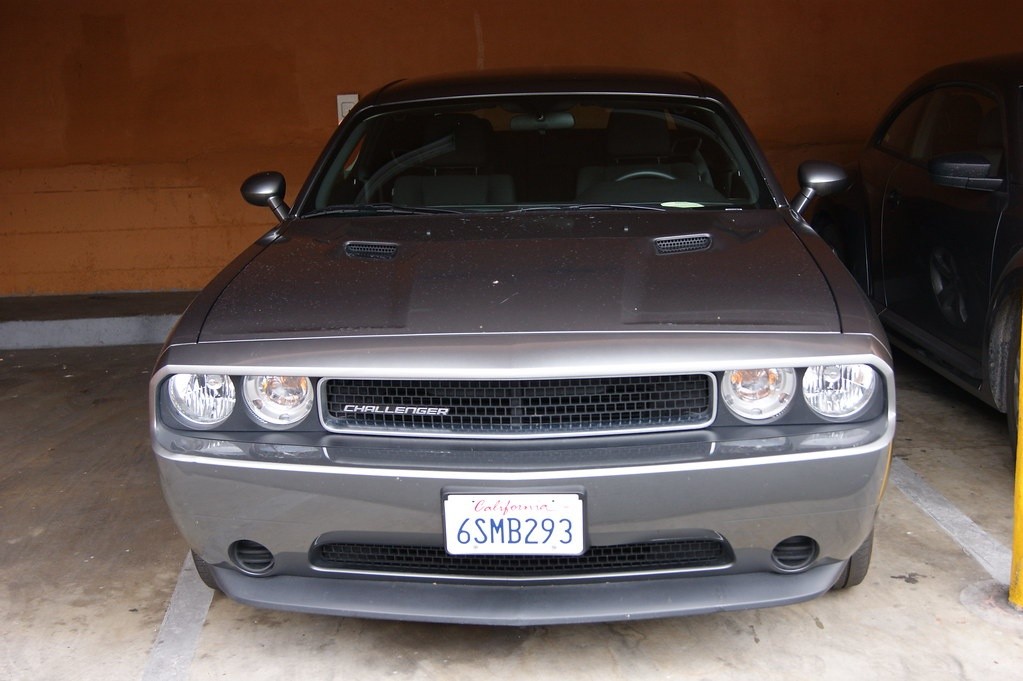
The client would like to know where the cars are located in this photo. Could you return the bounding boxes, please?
[146,64,897,629]
[809,52,1023,474]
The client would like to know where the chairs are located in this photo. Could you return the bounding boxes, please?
[376,114,515,206]
[575,108,706,205]
